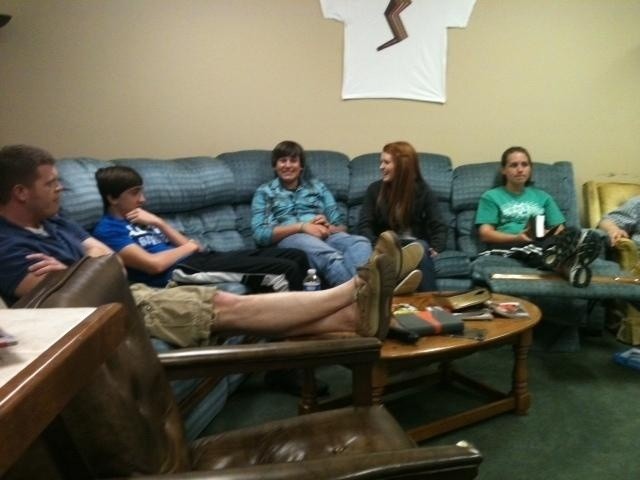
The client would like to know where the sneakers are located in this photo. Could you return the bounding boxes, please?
[394,269,422,297]
[542,226,580,269]
[355,254,393,340]
[369,231,404,281]
[568,229,602,288]
[399,241,425,279]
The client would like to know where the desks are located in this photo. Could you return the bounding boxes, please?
[0,291,131,480]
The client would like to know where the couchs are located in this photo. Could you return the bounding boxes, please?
[348,152,471,294]
[584,180,639,346]
[451,160,639,300]
[15,254,483,480]
[51,158,229,438]
[112,156,273,387]
[216,150,348,291]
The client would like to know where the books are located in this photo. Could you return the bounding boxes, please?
[390,310,465,339]
[452,300,531,321]
[522,214,546,240]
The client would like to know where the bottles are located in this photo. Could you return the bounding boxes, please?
[304,269,321,290]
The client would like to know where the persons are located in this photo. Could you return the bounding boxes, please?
[249,139,425,295]
[0,145,403,349]
[356,140,448,292]
[596,193,640,247]
[474,146,603,290]
[91,165,329,399]
